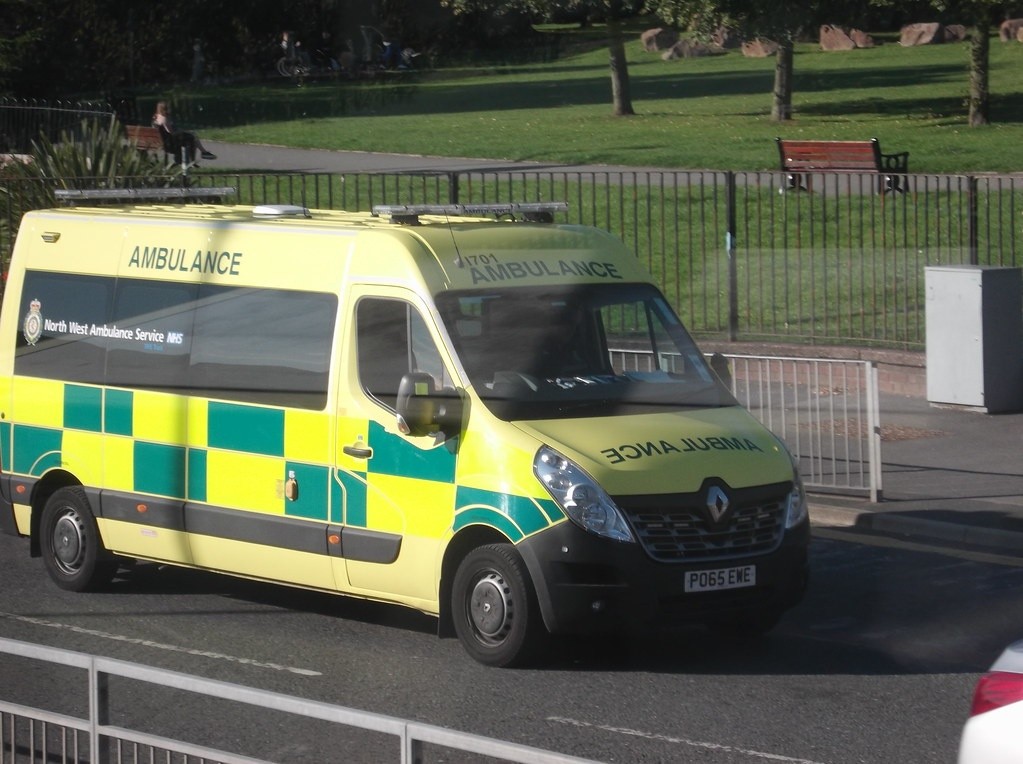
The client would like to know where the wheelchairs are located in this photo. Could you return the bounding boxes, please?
[276,49,318,78]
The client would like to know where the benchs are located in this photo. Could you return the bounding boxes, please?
[776,137,910,197]
[127,125,188,165]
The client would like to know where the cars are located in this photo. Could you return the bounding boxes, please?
[955,640,1023,764]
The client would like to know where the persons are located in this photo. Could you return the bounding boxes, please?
[152,102,217,169]
[541,317,614,380]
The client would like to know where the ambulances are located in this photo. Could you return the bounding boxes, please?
[0,204,812,667]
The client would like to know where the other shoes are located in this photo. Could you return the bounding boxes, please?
[191,164,200,169]
[200,150,217,159]
